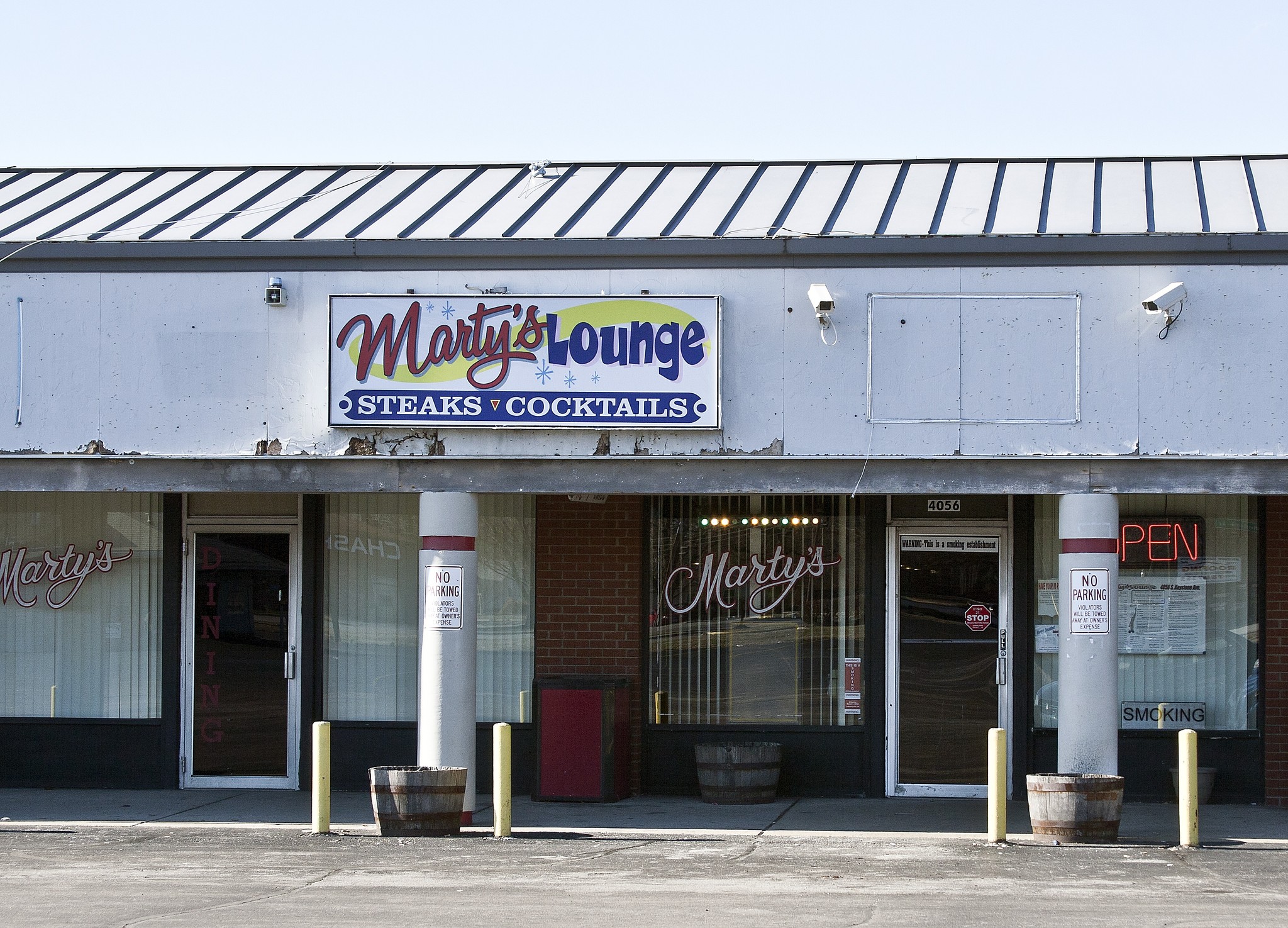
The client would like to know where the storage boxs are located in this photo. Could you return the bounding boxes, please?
[535,678,629,803]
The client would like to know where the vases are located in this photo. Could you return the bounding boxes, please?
[1169,767,1216,804]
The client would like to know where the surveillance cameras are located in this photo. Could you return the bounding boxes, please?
[808,281,835,323]
[1139,281,1187,315]
